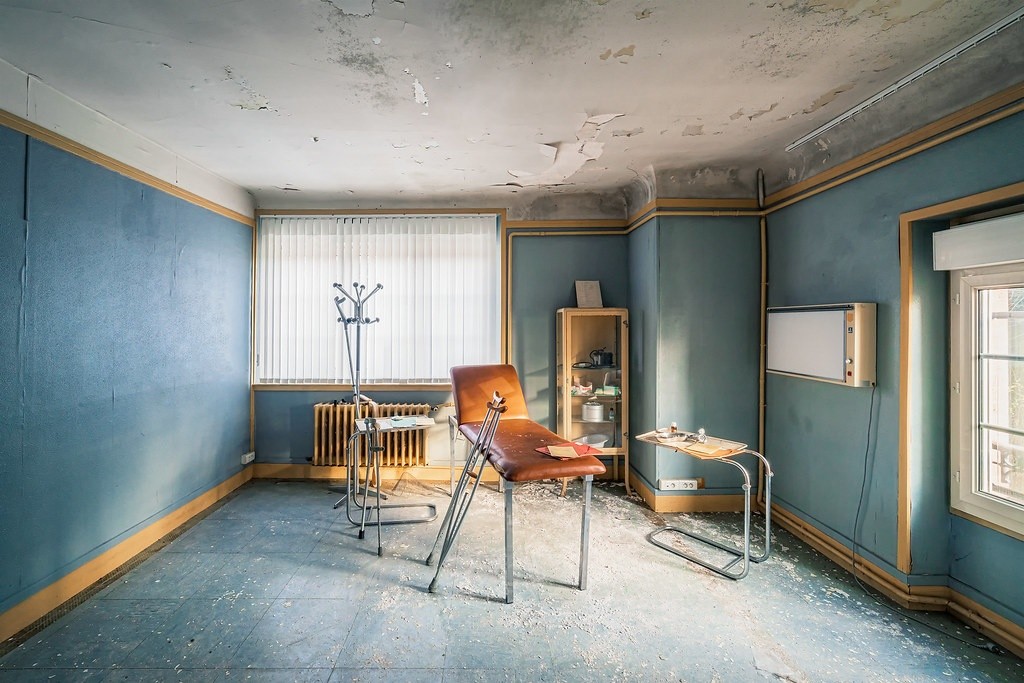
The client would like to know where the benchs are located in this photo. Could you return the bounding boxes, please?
[449,363,607,604]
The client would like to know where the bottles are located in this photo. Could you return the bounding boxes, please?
[669,421,678,435]
[697,428,707,443]
[609,408,615,421]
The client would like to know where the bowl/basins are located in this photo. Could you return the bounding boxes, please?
[573,434,609,448]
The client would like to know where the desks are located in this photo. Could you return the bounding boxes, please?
[634,427,774,579]
[345,414,439,525]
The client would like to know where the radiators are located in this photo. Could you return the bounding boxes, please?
[314,402,431,468]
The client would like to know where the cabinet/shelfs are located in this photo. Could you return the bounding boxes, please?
[556,307,634,500]
